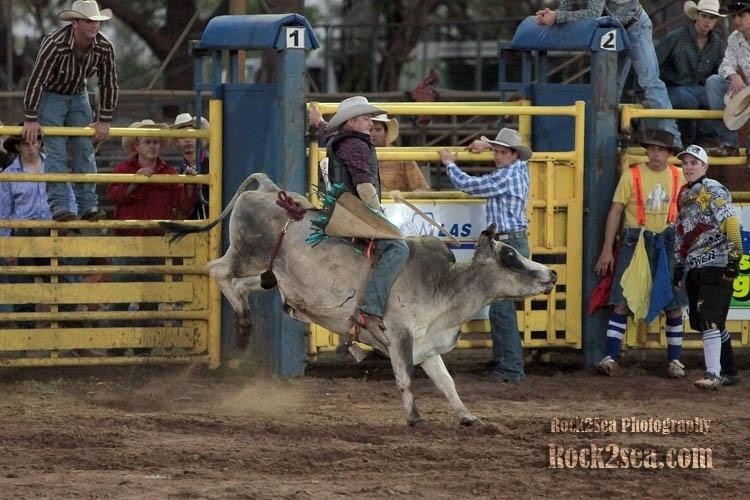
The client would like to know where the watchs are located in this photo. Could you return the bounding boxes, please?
[316,118,324,129]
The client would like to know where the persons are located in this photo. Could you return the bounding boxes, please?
[438,127,532,383]
[592,127,688,379]
[306,95,410,347]
[673,144,744,390]
[629,0,728,146]
[308,103,429,190]
[23,0,120,222]
[169,112,209,220]
[706,0,750,170]
[535,0,682,149]
[0,122,89,357]
[108,118,199,356]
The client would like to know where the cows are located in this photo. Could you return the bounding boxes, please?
[157,173,557,432]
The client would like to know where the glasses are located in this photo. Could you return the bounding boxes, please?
[724,1,745,12]
[136,137,161,147]
[487,144,512,154]
[17,139,41,148]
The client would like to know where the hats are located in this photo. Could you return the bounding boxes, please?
[323,94,387,133]
[369,113,400,146]
[2,122,44,154]
[480,127,533,161]
[719,0,750,17]
[169,112,210,149]
[675,143,710,166]
[638,128,683,156]
[56,1,113,22]
[120,119,169,154]
[683,1,730,21]
[722,85,750,132]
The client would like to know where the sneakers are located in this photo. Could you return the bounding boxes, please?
[349,308,392,347]
[720,370,742,387]
[471,361,528,383]
[591,355,619,377]
[666,357,686,380]
[707,142,742,159]
[693,373,724,391]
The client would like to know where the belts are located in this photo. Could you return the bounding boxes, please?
[624,6,644,30]
[494,230,529,241]
